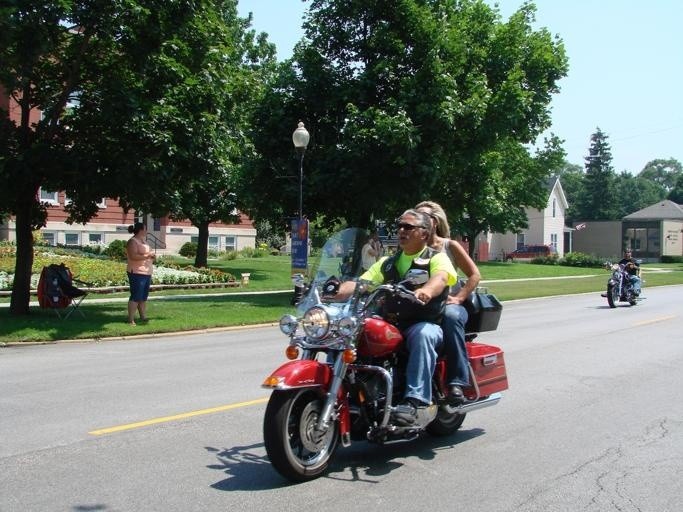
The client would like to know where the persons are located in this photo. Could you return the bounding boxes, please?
[415,200,482,402]
[600,248,641,302]
[360,235,380,274]
[124,221,157,327]
[337,255,353,282]
[290,208,458,427]
[375,240,384,262]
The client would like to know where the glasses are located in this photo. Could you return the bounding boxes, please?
[397,224,425,230]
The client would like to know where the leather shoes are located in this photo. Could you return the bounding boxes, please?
[394,401,419,426]
[449,385,465,403]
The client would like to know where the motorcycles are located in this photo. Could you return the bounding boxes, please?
[605,259,646,308]
[261,226,508,482]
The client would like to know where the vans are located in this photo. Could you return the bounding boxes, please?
[506,245,559,264]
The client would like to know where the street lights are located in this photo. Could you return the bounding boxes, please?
[292,118,310,220]
